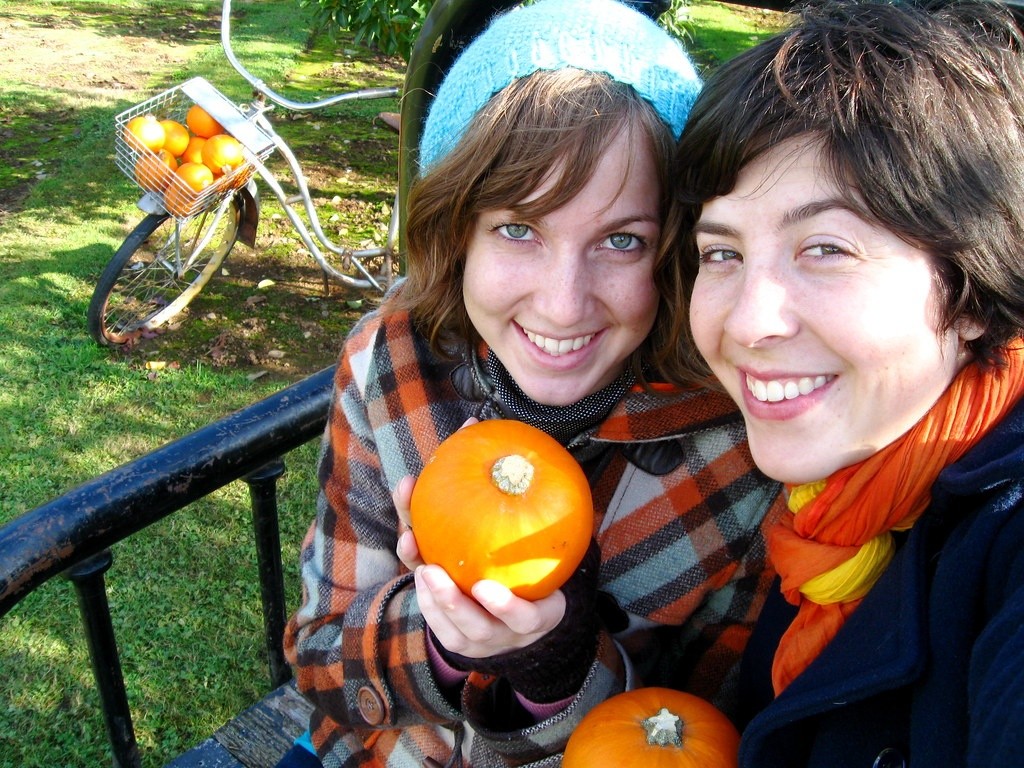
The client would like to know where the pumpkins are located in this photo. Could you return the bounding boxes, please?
[562,686,738,768]
[123,103,250,218]
[410,421,593,603]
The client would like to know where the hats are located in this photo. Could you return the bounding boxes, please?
[414,0,706,182]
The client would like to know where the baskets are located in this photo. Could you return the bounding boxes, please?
[114,77,280,225]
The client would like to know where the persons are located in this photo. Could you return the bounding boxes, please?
[661,0,1024,768]
[273,0,789,768]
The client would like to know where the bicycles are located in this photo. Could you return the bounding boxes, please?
[86,0,409,347]
